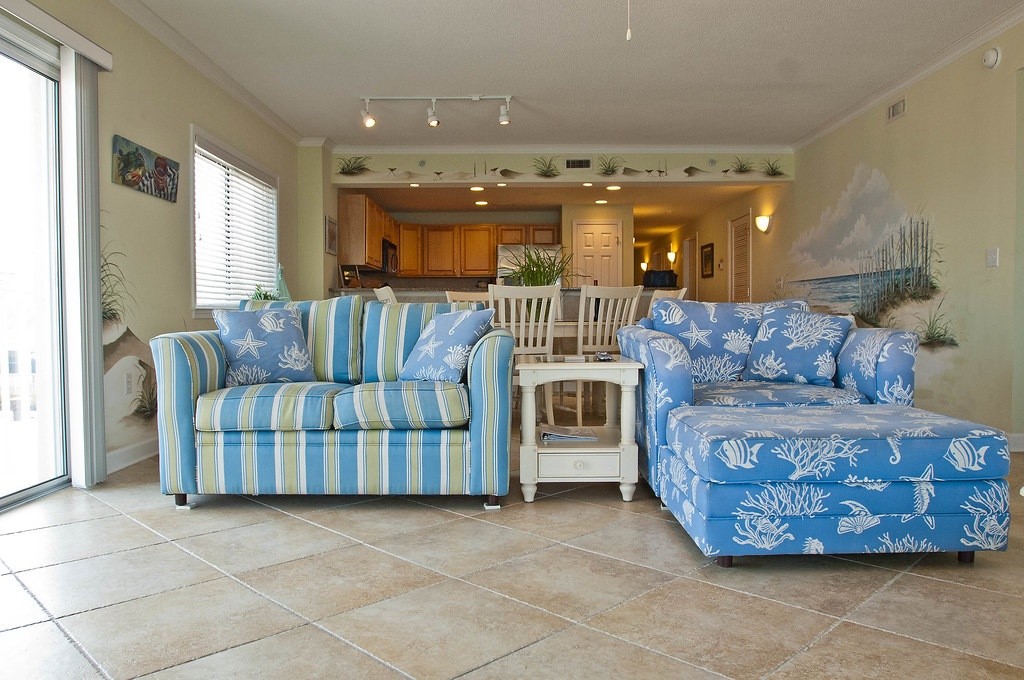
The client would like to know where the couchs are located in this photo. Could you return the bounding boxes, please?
[616,297,921,509]
[148,294,515,506]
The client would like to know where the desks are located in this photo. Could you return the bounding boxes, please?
[495,322,638,412]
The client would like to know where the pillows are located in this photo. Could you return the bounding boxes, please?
[212,306,318,388]
[398,307,496,383]
[740,305,854,388]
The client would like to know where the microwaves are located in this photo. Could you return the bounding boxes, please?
[382,239,398,273]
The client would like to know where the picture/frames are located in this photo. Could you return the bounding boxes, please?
[325,214,338,255]
[701,243,714,278]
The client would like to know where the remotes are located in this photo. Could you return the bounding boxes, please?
[596,353,612,361]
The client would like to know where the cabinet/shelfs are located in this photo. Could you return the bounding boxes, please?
[340,194,559,277]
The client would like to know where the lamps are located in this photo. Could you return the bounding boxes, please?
[499,97,511,125]
[755,215,772,234]
[360,99,375,128]
[667,252,677,264]
[426,99,440,128]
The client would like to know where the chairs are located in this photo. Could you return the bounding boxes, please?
[445,284,687,426]
[373,286,397,304]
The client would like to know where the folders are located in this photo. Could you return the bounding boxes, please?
[538,423,599,442]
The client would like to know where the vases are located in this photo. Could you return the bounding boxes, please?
[527,300,551,321]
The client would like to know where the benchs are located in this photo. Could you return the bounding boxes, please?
[658,404,1011,568]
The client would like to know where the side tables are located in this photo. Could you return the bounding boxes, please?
[515,355,644,502]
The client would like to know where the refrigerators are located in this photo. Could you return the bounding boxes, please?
[498,243,563,290]
